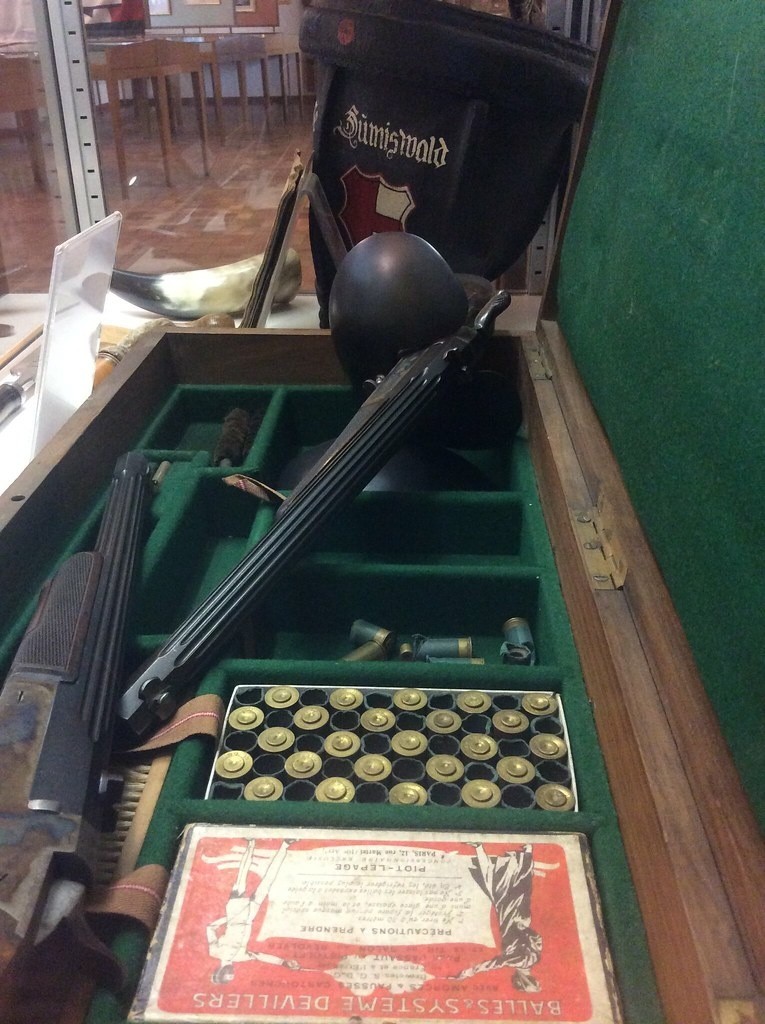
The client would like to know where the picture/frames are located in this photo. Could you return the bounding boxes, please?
[234,0,256,13]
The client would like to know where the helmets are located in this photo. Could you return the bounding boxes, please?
[327,232,465,385]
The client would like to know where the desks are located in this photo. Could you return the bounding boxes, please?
[0,31,304,200]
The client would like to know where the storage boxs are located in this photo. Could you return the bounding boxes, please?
[0,317,764,1024]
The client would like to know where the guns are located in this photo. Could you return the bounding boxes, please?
[113,286,514,741]
[1,449,154,1023]
